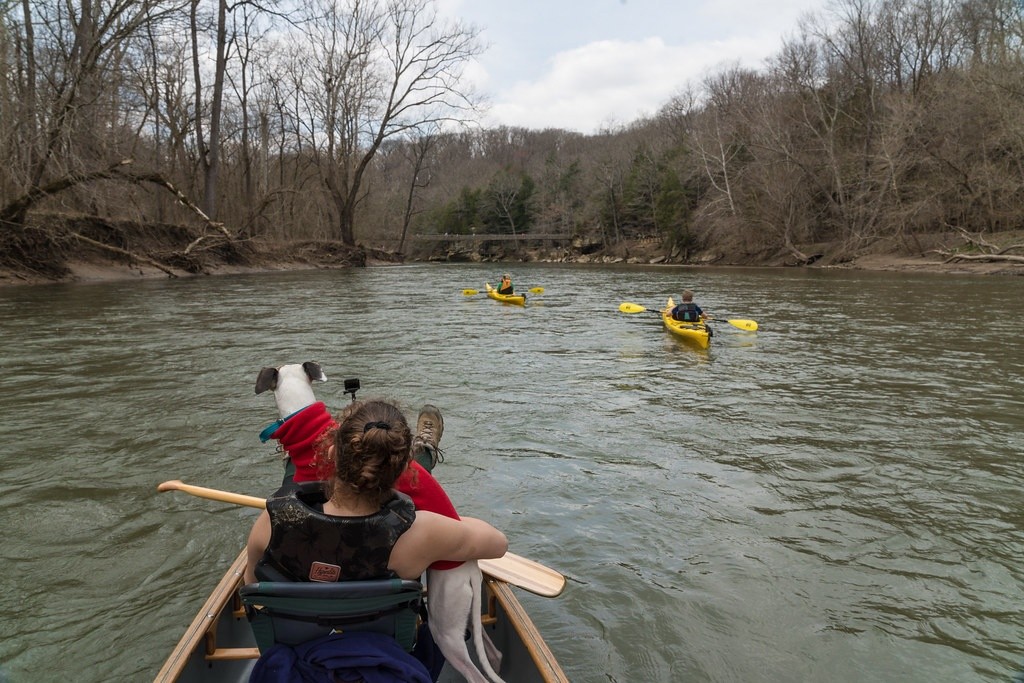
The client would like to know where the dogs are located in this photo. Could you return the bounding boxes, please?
[255,361,503,683]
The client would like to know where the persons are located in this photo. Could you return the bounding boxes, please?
[666,291,708,322]
[497,273,514,294]
[243,399,509,583]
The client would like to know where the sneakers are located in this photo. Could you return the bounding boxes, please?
[276,439,290,471]
[412,404,444,470]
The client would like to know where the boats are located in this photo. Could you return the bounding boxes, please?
[484,282,525,307]
[152,375,572,683]
[662,297,712,349]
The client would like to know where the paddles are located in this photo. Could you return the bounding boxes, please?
[619,302,758,330]
[157,481,566,599]
[463,287,543,295]
